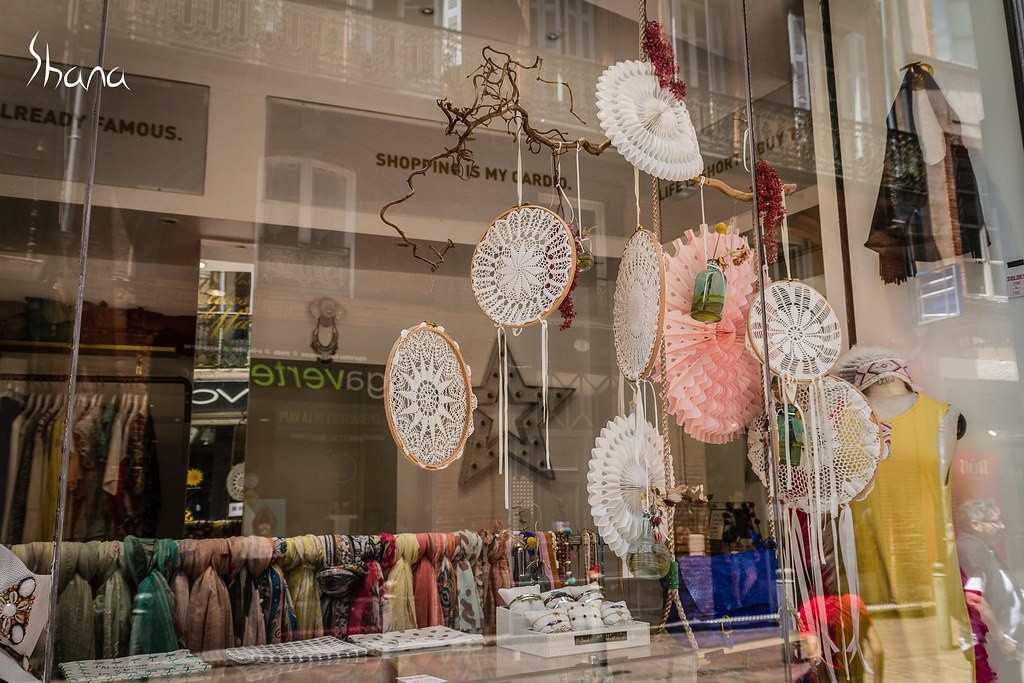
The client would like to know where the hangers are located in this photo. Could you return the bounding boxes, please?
[0,371,153,426]
